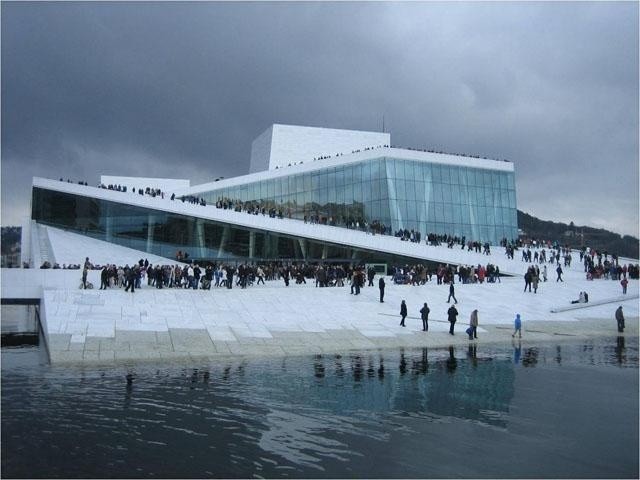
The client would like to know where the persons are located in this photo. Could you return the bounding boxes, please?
[447,281,458,304]
[436,262,500,285]
[57,177,292,221]
[378,275,386,303]
[390,262,432,286]
[399,300,407,327]
[346,264,376,293]
[512,313,522,339]
[201,264,265,290]
[447,305,459,335]
[146,263,201,291]
[302,208,492,259]
[0,260,80,270]
[176,250,189,261]
[469,310,479,340]
[615,306,625,332]
[263,262,345,287]
[312,342,542,383]
[499,230,640,304]
[420,303,431,331]
[79,256,149,292]
